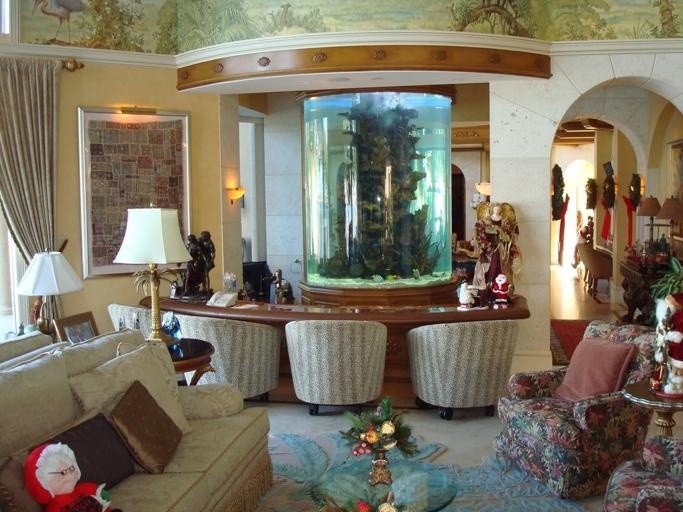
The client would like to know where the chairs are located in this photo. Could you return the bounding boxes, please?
[575,241,613,300]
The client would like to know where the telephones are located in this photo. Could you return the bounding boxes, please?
[206,291,238,307]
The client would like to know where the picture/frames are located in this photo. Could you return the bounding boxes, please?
[664,136,682,243]
[74,103,191,279]
[52,309,101,346]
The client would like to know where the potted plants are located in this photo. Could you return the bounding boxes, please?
[132,267,178,297]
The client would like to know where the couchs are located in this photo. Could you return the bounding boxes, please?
[171,315,282,400]
[282,316,390,416]
[491,318,662,500]
[108,302,186,385]
[405,316,520,422]
[0,328,274,511]
[601,434,682,512]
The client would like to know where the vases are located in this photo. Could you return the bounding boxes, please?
[367,448,394,512]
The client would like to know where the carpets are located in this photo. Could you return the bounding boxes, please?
[247,428,587,511]
[549,319,588,365]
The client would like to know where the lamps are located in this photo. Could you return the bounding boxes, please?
[225,185,246,206]
[16,246,84,337]
[111,200,195,344]
[635,196,661,273]
[653,193,682,256]
[474,180,490,197]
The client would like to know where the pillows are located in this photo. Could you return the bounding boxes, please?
[551,336,635,409]
[0,328,195,512]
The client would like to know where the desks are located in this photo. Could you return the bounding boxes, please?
[615,259,672,324]
[620,382,681,438]
[594,245,613,256]
[164,338,218,386]
[131,289,531,385]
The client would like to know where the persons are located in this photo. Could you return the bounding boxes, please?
[175,234,210,297]
[197,231,217,294]
[21,439,122,512]
[471,201,521,304]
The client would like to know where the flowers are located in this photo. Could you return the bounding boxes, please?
[337,394,422,461]
